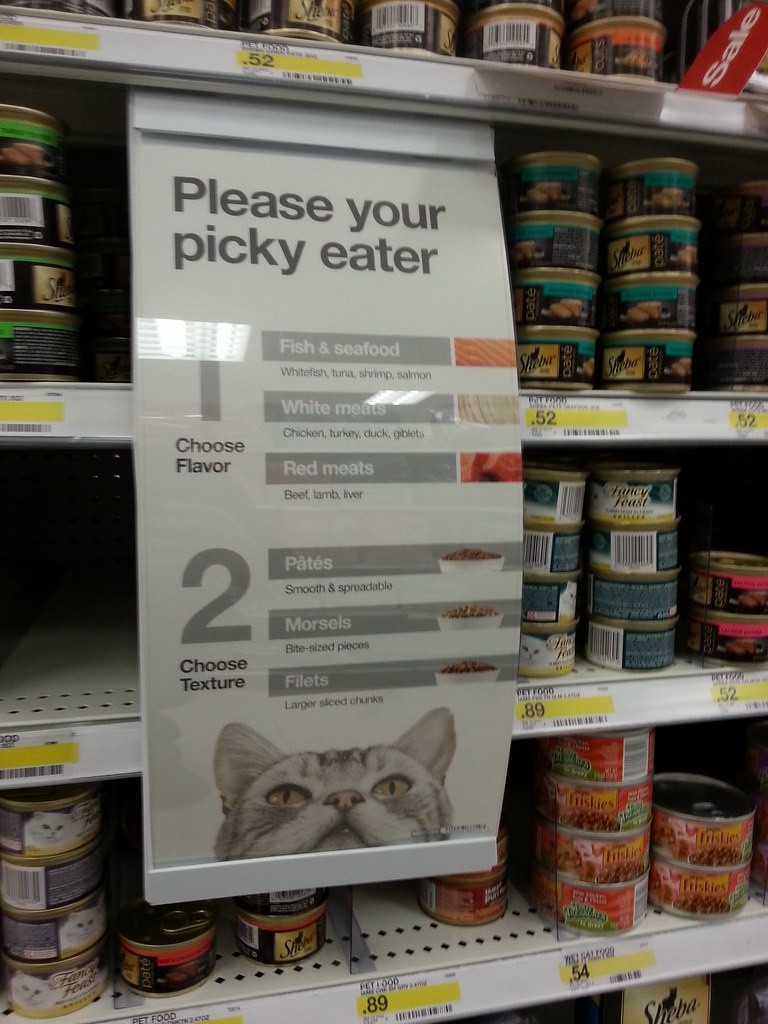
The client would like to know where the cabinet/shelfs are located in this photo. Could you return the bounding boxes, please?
[0,5,768,1024]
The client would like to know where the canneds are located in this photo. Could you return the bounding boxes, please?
[0,0,768,1020]
